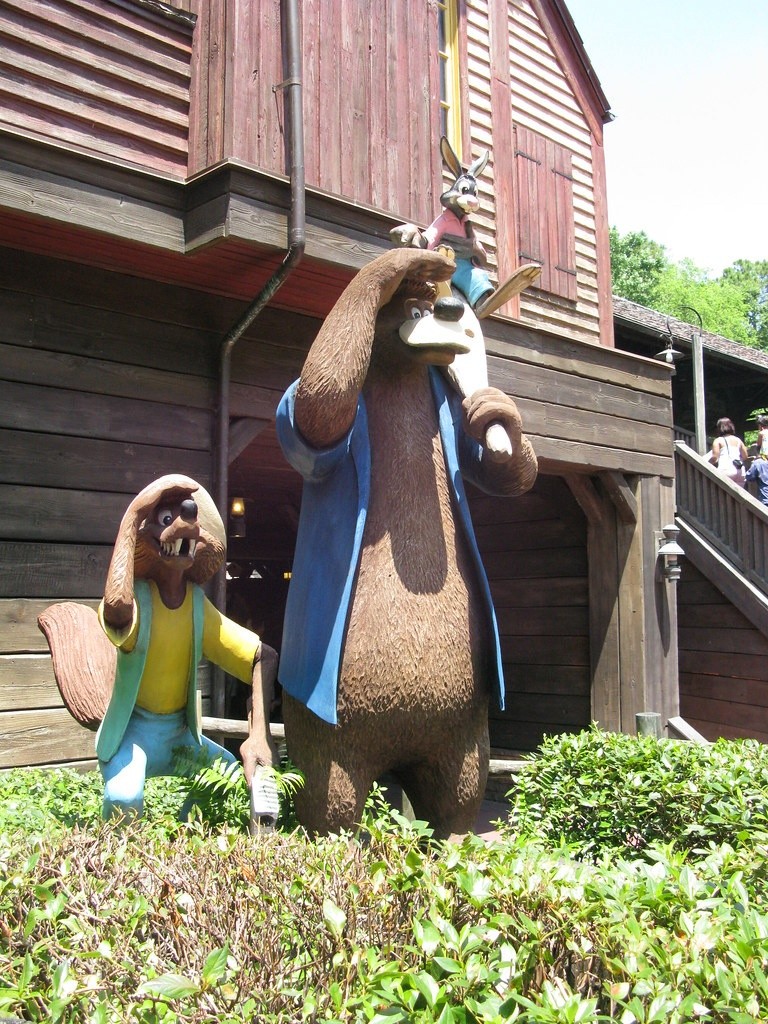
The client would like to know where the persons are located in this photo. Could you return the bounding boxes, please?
[755,415,768,461]
[708,417,749,489]
[745,446,768,506]
